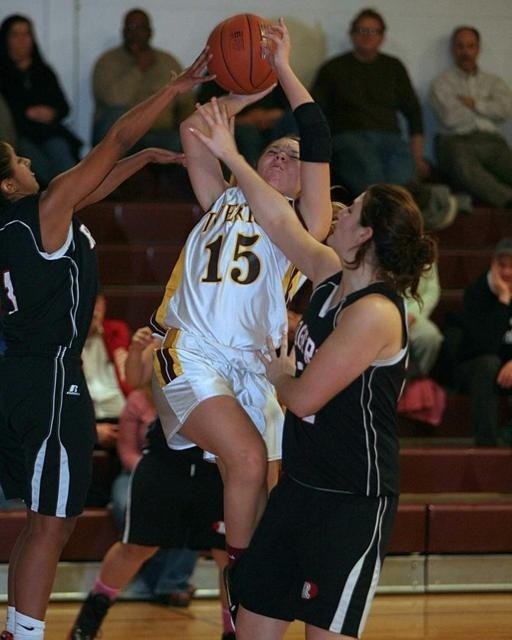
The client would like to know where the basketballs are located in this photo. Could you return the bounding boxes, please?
[205,14,281,95]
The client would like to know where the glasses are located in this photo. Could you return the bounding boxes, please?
[355,27,381,38]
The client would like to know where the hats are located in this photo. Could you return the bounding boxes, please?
[493,238,512,257]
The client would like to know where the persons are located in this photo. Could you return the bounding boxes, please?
[0,45,218,640]
[150,16,333,631]
[187,95,437,640]
[67,325,235,640]
[0,9,512,608]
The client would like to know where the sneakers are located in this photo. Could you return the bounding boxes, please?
[69,591,118,639]
[223,565,239,634]
[148,581,196,607]
